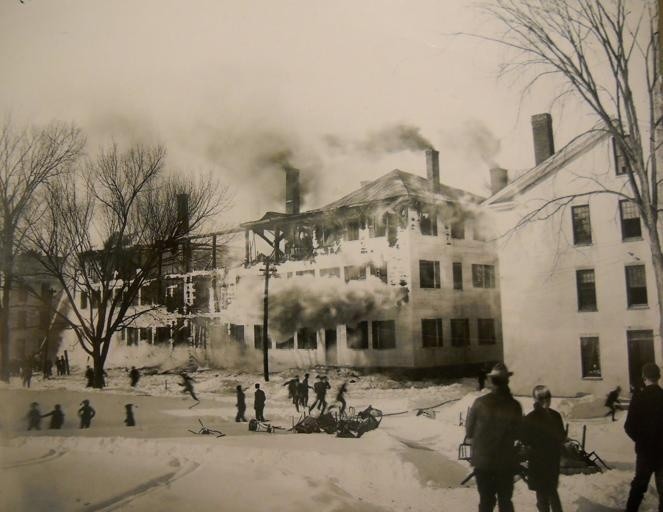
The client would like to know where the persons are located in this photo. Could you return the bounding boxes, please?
[0,351,66,389]
[253,384,267,421]
[176,373,199,400]
[42,403,65,429]
[604,386,621,422]
[622,362,663,511]
[128,366,141,387]
[279,372,350,413]
[477,367,487,390]
[123,403,135,426]
[24,401,43,430]
[234,385,248,422]
[465,361,523,512]
[523,384,563,512]
[101,369,108,387]
[84,365,93,389]
[77,399,96,429]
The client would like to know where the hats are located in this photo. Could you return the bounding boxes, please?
[485,362,513,379]
